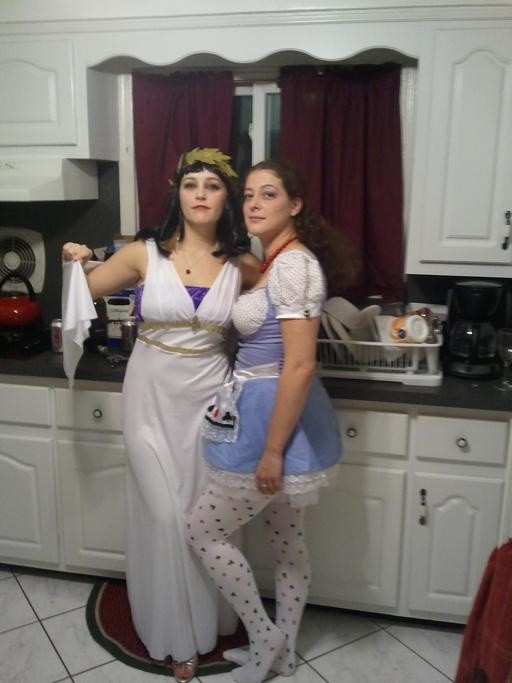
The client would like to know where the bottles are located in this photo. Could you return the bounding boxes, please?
[87,295,109,351]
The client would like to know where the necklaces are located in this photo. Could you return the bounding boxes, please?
[174,236,219,274]
[255,235,305,274]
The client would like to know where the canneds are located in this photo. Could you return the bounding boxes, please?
[50,318,62,354]
[121,321,137,352]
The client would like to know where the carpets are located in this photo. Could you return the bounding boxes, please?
[81,580,256,677]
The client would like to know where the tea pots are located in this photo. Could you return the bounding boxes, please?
[0,272,48,328]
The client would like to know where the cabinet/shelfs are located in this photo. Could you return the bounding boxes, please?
[402,4,512,283]
[0,377,61,581]
[241,400,404,629]
[59,379,145,587]
[404,415,512,631]
[1,21,118,165]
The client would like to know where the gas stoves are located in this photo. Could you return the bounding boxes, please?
[1,323,47,356]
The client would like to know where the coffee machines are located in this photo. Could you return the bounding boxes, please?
[442,280,505,381]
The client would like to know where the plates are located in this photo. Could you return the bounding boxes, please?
[321,307,401,361]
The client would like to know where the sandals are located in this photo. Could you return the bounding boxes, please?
[171,656,200,683]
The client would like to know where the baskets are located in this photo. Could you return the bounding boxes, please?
[314,329,445,388]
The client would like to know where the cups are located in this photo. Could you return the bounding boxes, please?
[385,313,428,353]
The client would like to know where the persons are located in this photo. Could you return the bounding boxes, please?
[61,158,268,683]
[185,158,323,683]
